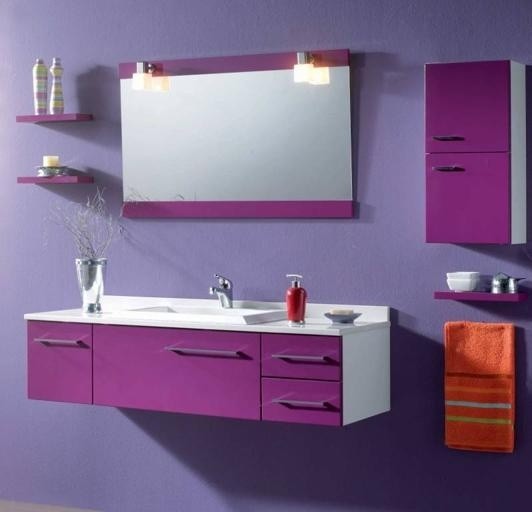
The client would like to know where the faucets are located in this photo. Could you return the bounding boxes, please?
[210,275,235,308]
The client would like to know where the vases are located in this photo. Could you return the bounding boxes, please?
[75,256,107,313]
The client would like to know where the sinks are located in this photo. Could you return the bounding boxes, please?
[123,305,289,325]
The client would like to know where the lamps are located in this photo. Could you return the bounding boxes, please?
[131,60,155,91]
[291,51,315,84]
[149,64,168,92]
[310,54,330,86]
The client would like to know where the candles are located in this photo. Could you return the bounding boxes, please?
[40,154,58,167]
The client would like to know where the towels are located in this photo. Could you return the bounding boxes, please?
[443,320,515,454]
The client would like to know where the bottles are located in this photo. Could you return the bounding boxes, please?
[32,55,66,116]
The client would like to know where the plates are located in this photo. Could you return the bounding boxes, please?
[326,313,363,322]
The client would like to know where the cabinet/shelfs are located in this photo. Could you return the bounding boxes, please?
[256,324,391,428]
[89,322,260,423]
[24,320,90,405]
[421,60,527,247]
[15,112,95,186]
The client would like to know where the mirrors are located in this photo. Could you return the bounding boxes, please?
[117,47,357,218]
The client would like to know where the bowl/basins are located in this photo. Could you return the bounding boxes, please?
[446,272,479,293]
